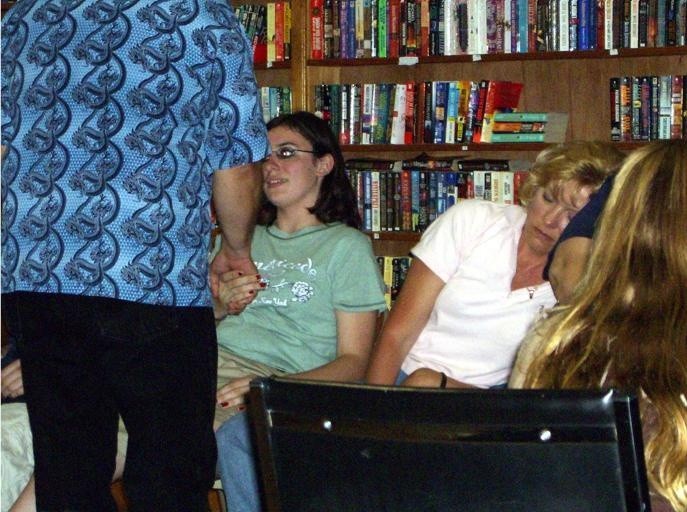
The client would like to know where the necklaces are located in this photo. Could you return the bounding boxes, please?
[516,243,546,300]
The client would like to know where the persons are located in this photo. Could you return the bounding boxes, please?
[1,316,35,512]
[542,173,615,305]
[507,139,686,511]
[9,111,387,512]
[2,2,269,512]
[215,140,627,512]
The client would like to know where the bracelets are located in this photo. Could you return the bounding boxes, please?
[440,372,447,388]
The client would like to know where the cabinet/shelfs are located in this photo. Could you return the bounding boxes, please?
[230,0,687,339]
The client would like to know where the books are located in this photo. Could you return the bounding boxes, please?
[227,2,686,63]
[374,256,414,318]
[609,75,686,138]
[264,74,568,145]
[345,156,529,231]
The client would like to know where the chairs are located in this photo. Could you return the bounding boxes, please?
[249,374,648,512]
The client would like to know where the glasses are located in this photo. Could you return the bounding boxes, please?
[261,147,317,162]
